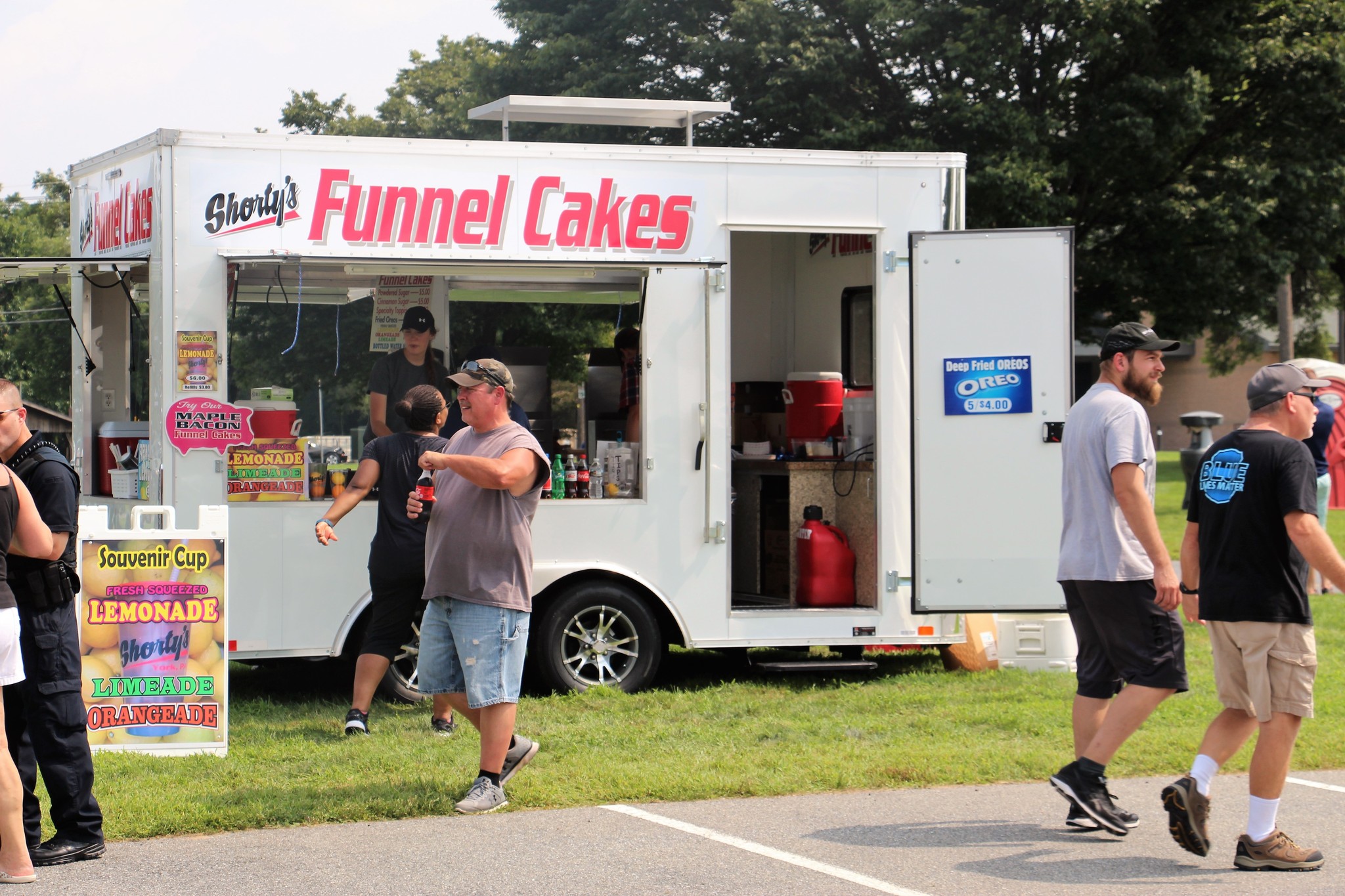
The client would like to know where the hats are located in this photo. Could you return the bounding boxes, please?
[1246,363,1332,411]
[1098,321,1180,362]
[399,306,435,333]
[444,359,514,393]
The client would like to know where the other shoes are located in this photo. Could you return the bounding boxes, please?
[25,835,41,850]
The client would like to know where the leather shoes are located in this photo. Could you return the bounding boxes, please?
[28,830,107,867]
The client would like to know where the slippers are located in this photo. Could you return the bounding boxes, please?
[0,870,36,883]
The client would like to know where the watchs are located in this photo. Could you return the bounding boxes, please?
[1179,581,1199,595]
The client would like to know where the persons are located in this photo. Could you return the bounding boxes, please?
[1049,321,1188,837]
[406,359,551,816]
[1162,362,1345,871]
[0,378,106,885]
[314,307,642,740]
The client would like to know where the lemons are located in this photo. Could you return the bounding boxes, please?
[607,483,620,495]
[331,472,345,485]
[79,540,225,746]
[177,331,218,390]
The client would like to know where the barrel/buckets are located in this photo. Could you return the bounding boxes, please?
[97,421,149,496]
[795,505,855,607]
[843,391,877,460]
[234,400,302,438]
[781,372,847,455]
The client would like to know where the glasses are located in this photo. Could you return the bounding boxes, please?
[1292,392,1316,403]
[438,401,451,413]
[0,408,19,421]
[460,360,505,387]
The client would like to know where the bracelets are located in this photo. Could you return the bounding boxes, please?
[316,519,333,528]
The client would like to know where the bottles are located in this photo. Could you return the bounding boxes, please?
[608,442,635,496]
[564,455,577,498]
[604,443,611,484]
[552,454,565,500]
[577,455,589,498]
[589,458,603,499]
[118,446,139,470]
[414,469,434,523]
[541,453,552,499]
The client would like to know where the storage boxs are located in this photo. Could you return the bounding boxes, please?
[841,396,881,464]
[992,616,1080,676]
[231,385,303,440]
[105,464,139,502]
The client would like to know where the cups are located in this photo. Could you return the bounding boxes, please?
[308,463,327,501]
[114,581,194,736]
[186,344,207,384]
[329,469,348,501]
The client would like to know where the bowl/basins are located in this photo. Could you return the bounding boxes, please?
[805,442,843,455]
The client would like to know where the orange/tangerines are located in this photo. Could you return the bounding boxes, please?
[309,472,325,498]
[332,485,345,499]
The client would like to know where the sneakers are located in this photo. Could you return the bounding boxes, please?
[1066,796,1140,829]
[1161,772,1212,857]
[1049,761,1128,836]
[1233,822,1325,872]
[499,735,540,786]
[453,776,509,814]
[345,708,371,736]
[431,711,458,737]
